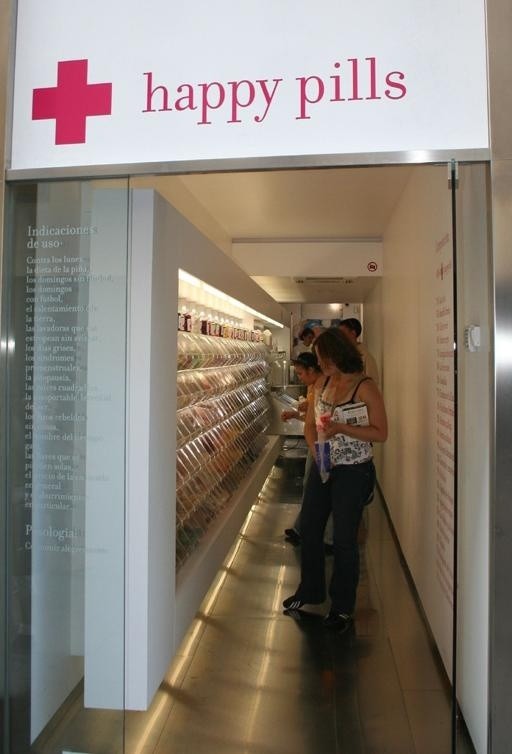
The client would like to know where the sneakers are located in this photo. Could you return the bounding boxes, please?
[284,528,296,537]
[283,594,324,609]
[324,608,352,634]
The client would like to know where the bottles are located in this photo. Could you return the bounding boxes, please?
[178,306,264,342]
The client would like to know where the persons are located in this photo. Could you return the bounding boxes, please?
[283,318,386,637]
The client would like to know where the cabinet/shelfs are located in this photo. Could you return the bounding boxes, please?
[84,186,290,714]
